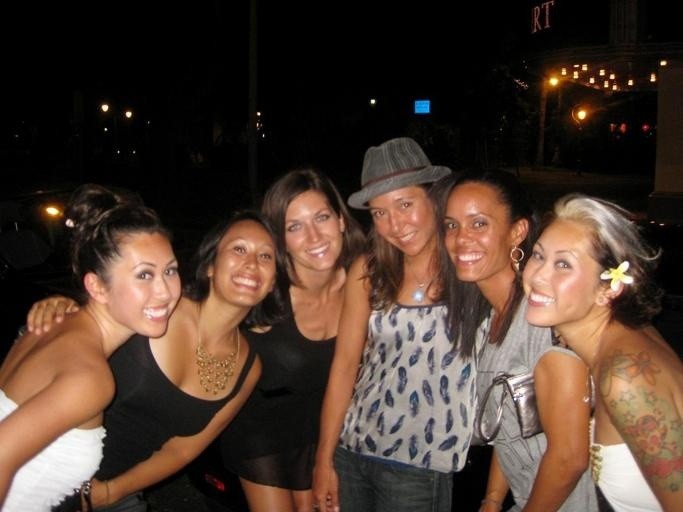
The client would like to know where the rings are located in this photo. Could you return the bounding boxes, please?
[312,503,320,511]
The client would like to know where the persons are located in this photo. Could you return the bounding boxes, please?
[25,209,290,511]
[524,188,682,512]
[0,183,185,511]
[231,167,374,512]
[437,167,602,512]
[311,136,496,512]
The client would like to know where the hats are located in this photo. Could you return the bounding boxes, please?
[347,137,452,212]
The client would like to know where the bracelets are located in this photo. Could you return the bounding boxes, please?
[100,480,116,509]
[80,481,98,512]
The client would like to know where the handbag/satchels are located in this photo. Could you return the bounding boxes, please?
[478,373,594,446]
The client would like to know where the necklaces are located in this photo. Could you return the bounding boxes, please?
[196,307,242,397]
[582,332,605,404]
[403,254,435,303]
